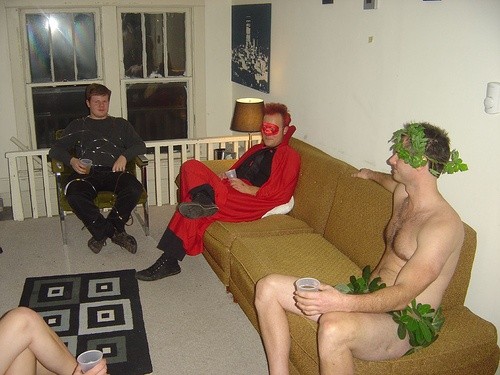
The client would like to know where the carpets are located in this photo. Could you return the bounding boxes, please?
[17,265,156,375]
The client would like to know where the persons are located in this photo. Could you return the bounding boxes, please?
[134,102,302,282]
[256,122,466,375]
[0,306,110,375]
[46,83,146,255]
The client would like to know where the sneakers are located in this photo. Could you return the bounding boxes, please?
[112,230,137,255]
[86,234,110,254]
[134,250,182,282]
[178,191,218,220]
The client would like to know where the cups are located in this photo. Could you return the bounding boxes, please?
[80,158,92,174]
[225,169,237,179]
[295,278,320,292]
[77,349,104,374]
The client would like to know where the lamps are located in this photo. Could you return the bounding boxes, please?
[231,96,266,152]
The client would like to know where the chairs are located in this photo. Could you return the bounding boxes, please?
[45,128,152,237]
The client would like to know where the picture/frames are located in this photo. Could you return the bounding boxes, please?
[363,0,377,11]
[231,3,272,94]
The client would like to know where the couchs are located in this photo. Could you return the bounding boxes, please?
[180,136,500,374]
